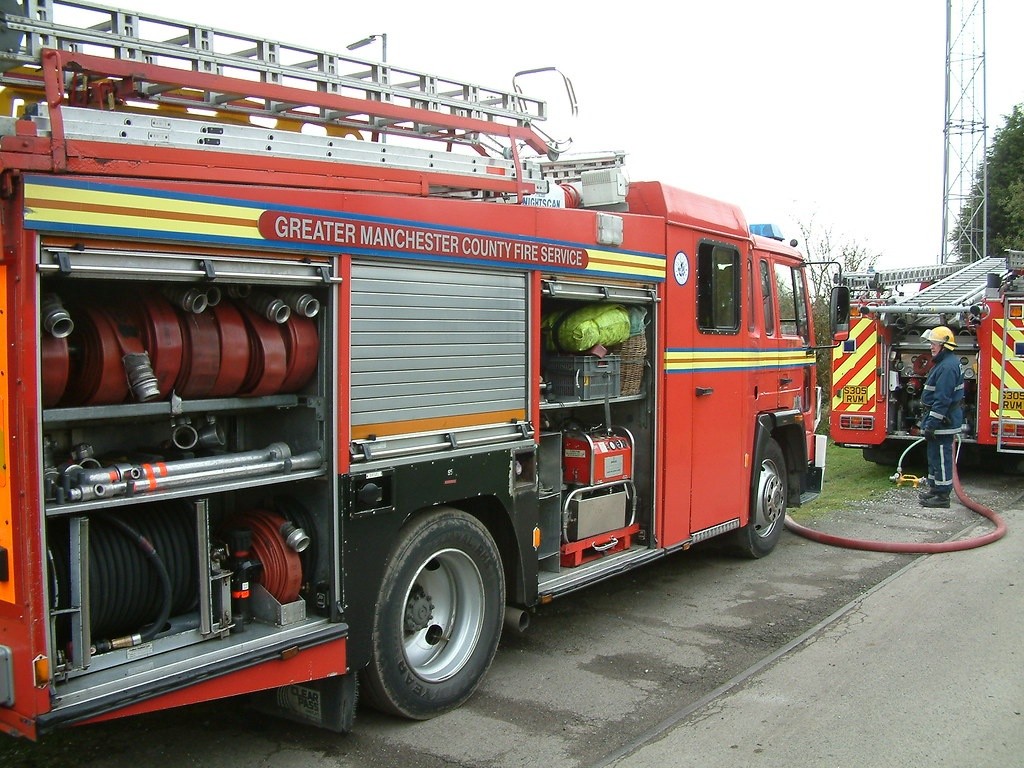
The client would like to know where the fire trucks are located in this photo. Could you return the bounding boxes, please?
[0,0,832,745]
[827,245,1024,471]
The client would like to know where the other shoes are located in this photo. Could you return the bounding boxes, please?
[919,496,951,508]
[919,492,934,499]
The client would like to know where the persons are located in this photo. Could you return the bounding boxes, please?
[917,326,963,509]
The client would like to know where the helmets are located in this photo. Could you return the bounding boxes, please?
[920,325,959,347]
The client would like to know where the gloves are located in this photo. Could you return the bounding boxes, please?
[925,427,937,442]
